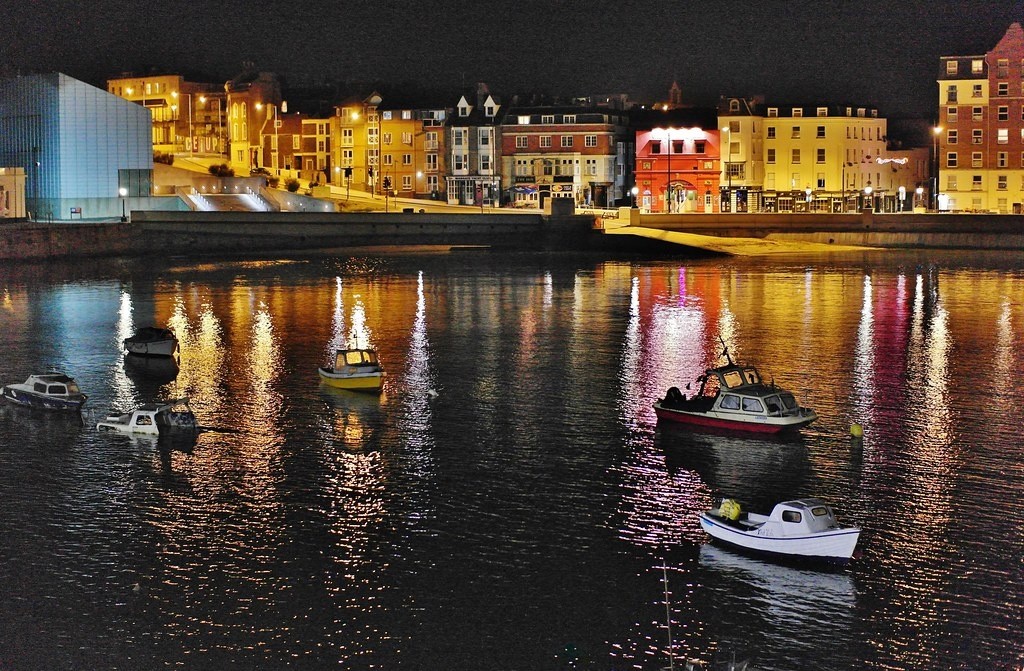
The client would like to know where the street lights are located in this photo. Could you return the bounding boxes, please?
[255,102,279,177]
[118,187,129,223]
[721,124,733,212]
[334,165,355,200]
[350,105,383,196]
[171,89,193,159]
[653,127,672,213]
[931,118,945,210]
[199,96,223,162]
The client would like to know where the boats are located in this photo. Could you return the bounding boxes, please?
[316,344,386,393]
[123,322,180,359]
[96,396,200,444]
[4,366,90,411]
[697,493,865,569]
[648,330,820,437]
